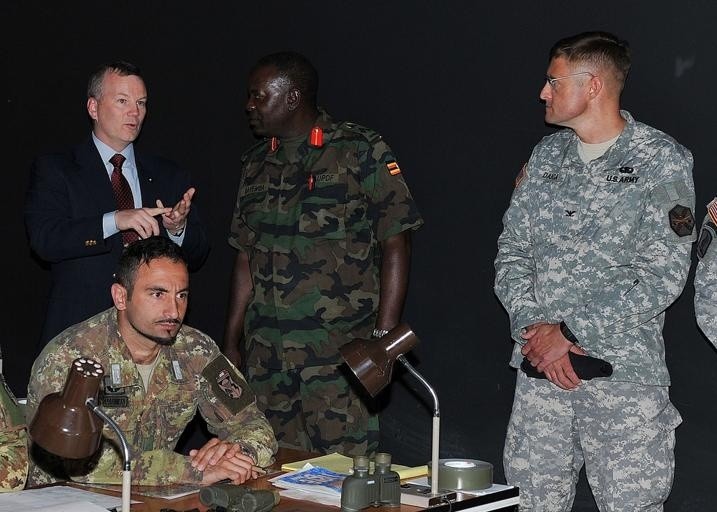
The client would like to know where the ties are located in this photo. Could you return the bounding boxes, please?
[109,154,140,246]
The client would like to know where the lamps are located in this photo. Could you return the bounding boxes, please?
[27,356,131,512]
[339,323,457,507]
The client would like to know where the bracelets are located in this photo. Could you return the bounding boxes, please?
[559,320,577,345]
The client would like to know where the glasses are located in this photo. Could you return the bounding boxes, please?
[547,72,594,90]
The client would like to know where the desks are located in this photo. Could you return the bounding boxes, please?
[1,447,521,512]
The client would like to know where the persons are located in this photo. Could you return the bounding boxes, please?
[0,370,28,494]
[694,196,717,347]
[28,236,278,488]
[16,61,210,354]
[222,51,424,459]
[492,40,697,512]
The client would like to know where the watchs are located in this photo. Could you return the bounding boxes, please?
[372,327,391,339]
